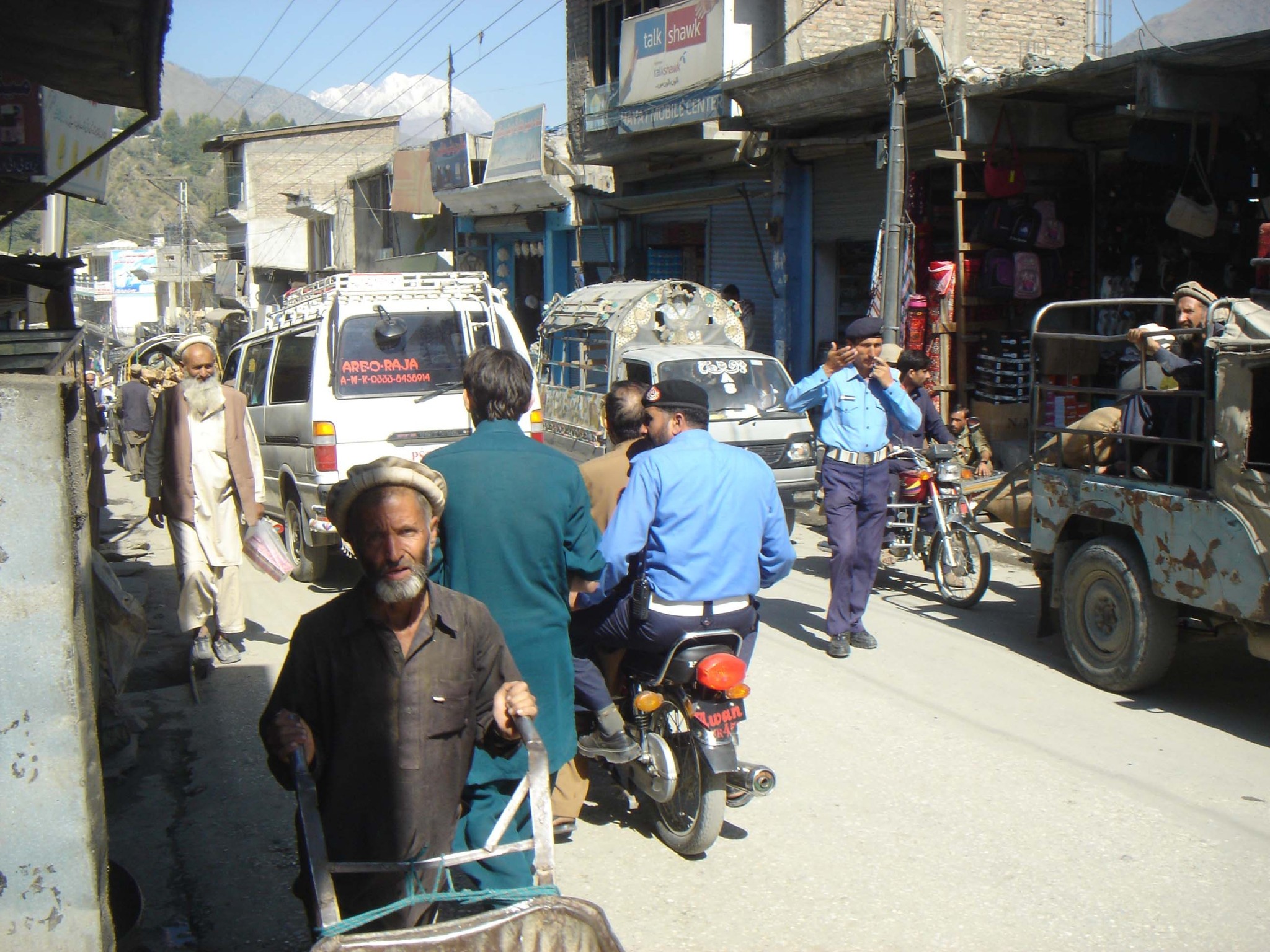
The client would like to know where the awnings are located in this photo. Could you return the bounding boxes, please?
[0,0,174,231]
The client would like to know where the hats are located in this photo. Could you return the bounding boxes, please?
[101,379,112,387]
[326,457,446,538]
[130,364,141,374]
[175,333,217,361]
[846,317,884,339]
[85,369,95,375]
[1173,281,1218,306]
[641,379,709,410]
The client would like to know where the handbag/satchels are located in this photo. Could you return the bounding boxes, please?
[243,519,293,582]
[1166,158,1217,237]
[983,157,1024,197]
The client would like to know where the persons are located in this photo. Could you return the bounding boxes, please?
[144,333,265,663]
[1112,281,1218,482]
[708,361,779,410]
[722,284,756,350]
[420,346,605,909]
[116,363,156,482]
[259,456,539,943]
[552,380,796,836]
[785,318,922,657]
[946,404,994,476]
[84,369,114,465]
[879,349,966,588]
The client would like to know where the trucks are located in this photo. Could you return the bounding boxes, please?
[97,332,189,472]
[528,277,827,537]
[957,297,1270,695]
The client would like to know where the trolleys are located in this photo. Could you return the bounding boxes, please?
[285,716,628,952]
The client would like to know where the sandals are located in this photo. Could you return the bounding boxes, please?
[552,813,576,834]
[726,786,751,806]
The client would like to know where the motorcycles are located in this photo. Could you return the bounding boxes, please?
[813,433,992,611]
[577,627,778,857]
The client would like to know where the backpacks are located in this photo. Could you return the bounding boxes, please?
[971,199,1064,299]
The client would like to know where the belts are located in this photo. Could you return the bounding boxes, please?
[649,592,751,617]
[826,445,887,466]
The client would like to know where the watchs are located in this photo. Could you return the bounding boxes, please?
[979,461,988,465]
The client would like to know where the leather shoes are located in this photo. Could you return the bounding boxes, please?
[575,727,644,763]
[829,632,850,656]
[846,628,877,649]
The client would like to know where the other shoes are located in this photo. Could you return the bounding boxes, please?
[130,474,141,481]
[192,635,214,666]
[215,639,243,665]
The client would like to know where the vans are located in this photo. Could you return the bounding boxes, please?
[219,269,545,585]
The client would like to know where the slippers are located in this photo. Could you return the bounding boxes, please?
[932,570,966,588]
[879,551,895,566]
[1133,466,1156,480]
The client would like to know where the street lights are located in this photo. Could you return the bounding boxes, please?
[180,238,199,334]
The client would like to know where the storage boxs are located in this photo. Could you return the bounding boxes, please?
[1044,373,1089,427]
[970,398,1044,441]
